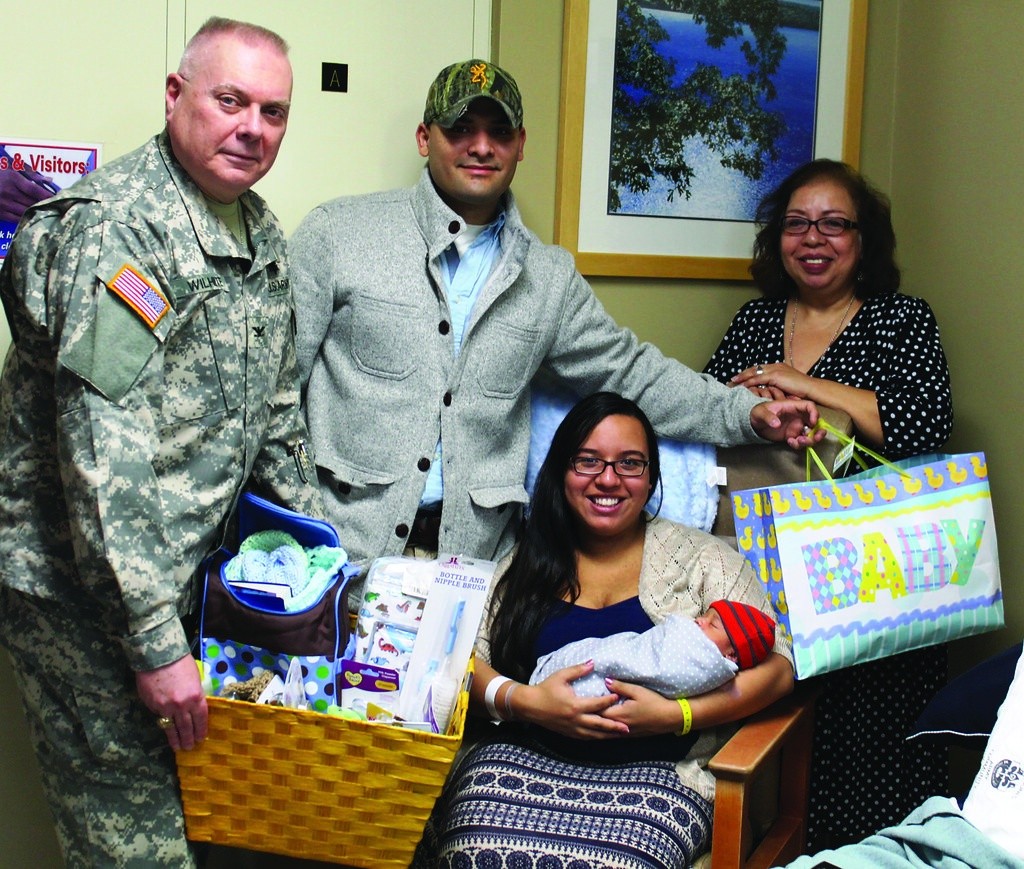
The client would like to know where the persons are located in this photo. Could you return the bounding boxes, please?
[284,60,826,610]
[0,160,55,222]
[527,599,776,709]
[438,391,796,869]
[702,157,954,856]
[0,15,324,869]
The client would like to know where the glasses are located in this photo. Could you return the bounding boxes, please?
[779,215,862,236]
[568,457,654,477]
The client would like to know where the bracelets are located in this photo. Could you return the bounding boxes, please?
[485,675,522,727]
[675,697,692,737]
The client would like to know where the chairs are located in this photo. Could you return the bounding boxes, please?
[524,385,851,869]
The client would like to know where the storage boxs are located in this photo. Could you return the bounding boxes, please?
[176,613,475,869]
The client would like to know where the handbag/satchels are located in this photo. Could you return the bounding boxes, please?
[731,418,1009,681]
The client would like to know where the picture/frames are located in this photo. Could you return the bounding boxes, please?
[553,0,867,280]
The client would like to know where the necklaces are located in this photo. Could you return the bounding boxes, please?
[790,292,854,378]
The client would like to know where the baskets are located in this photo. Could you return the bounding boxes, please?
[171,651,474,869]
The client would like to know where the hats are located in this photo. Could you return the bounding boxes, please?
[423,59,523,131]
[709,599,777,669]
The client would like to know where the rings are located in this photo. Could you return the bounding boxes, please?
[758,384,765,389]
[157,718,173,729]
[754,362,758,367]
[756,365,764,376]
[801,424,810,435]
